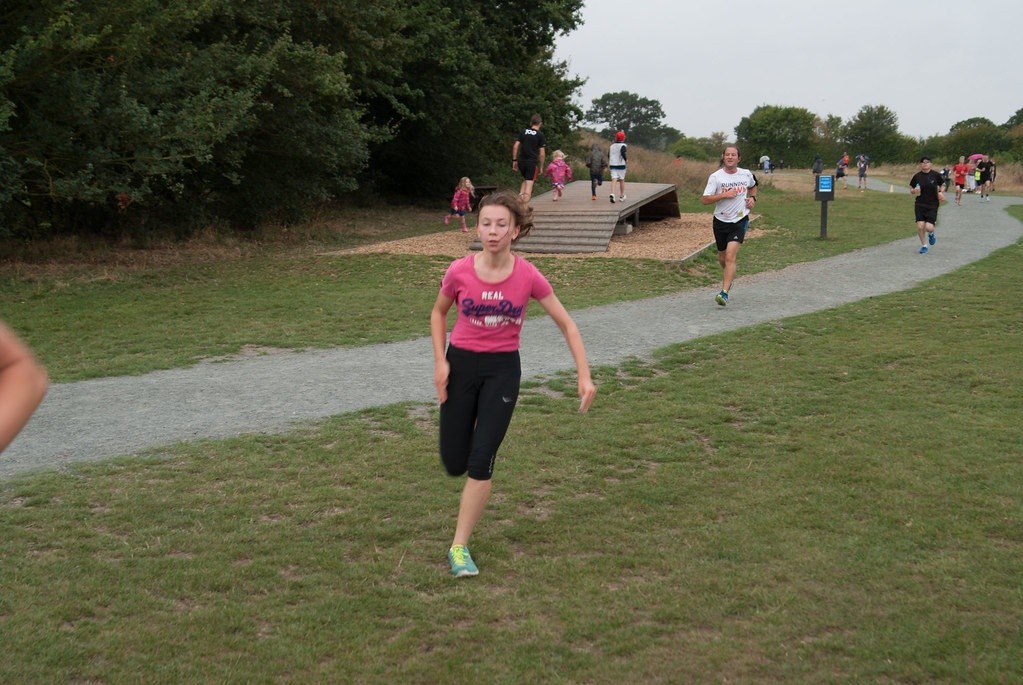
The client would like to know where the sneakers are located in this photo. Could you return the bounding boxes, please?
[920,247,928,255]
[716,290,727,307]
[448,544,478,578]
[929,231,936,246]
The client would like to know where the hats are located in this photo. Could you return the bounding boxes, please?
[920,157,932,164]
[616,132,625,142]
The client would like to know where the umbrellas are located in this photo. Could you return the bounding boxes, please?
[968,154,983,160]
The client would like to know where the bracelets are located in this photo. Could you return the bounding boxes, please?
[751,195,756,201]
[938,191,941,192]
[512,159,517,161]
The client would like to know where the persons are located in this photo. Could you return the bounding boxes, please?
[835,152,850,189]
[432,194,598,577]
[584,143,608,201]
[811,155,823,192]
[940,164,951,191]
[443,177,473,232]
[953,156,968,206]
[674,155,683,167]
[0,324,47,460]
[909,157,945,255]
[966,154,996,203]
[546,150,572,201]
[513,114,546,200]
[702,146,759,307]
[857,155,868,189]
[608,132,630,202]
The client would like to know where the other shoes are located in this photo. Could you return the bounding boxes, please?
[445,216,449,225]
[553,197,558,201]
[620,195,626,202]
[592,195,598,200]
[610,194,615,202]
[558,186,562,196]
[981,197,985,204]
[462,224,469,232]
[985,196,990,203]
